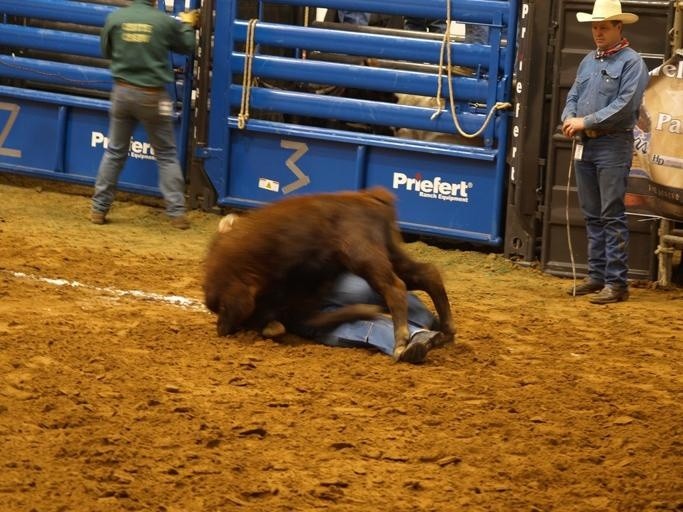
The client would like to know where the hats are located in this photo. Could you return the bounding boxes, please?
[573,1,639,25]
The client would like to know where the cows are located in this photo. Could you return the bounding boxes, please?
[198,185,458,367]
[258,56,488,149]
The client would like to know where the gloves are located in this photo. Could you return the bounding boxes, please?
[177,9,199,27]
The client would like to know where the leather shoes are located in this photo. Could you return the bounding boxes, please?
[566,280,604,296]
[588,286,628,305]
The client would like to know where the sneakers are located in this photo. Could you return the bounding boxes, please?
[399,329,445,363]
[85,207,105,223]
[171,219,190,230]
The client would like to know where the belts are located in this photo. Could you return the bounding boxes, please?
[582,127,633,139]
[114,78,164,95]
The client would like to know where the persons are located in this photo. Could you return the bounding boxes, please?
[215,207,449,363]
[559,0,650,303]
[254,9,491,137]
[85,0,201,231]
